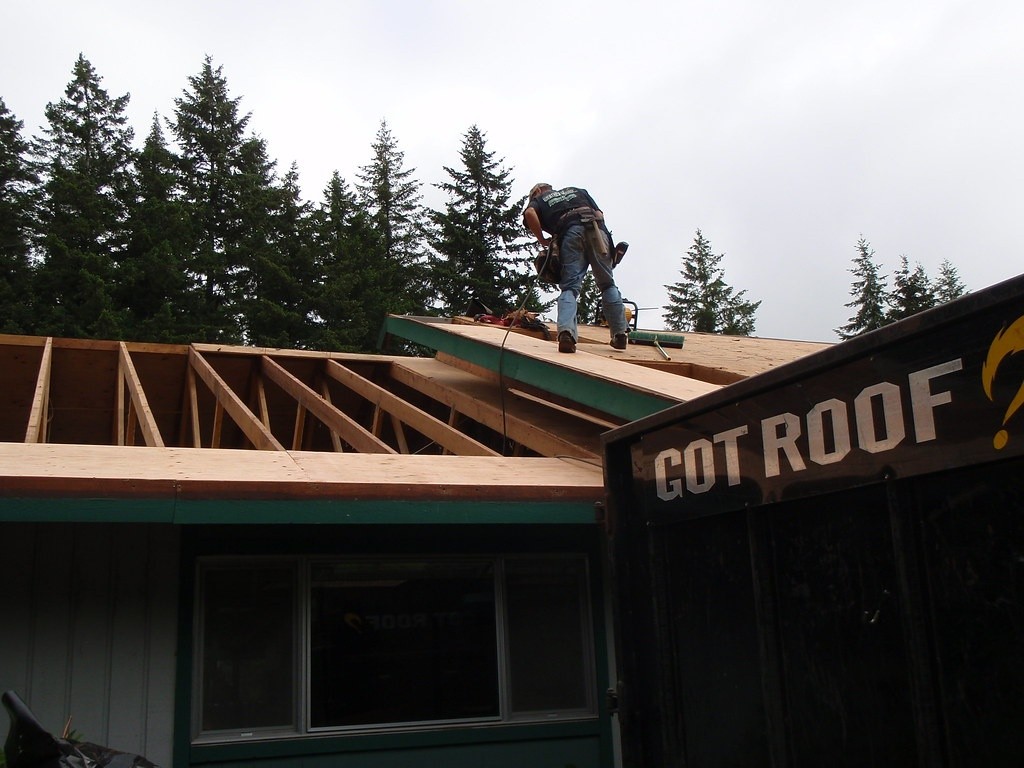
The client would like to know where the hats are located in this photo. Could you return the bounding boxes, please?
[529,183,552,201]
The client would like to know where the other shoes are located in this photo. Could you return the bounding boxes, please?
[610,334,628,349]
[559,332,576,353]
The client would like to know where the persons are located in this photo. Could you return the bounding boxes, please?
[524,183,628,353]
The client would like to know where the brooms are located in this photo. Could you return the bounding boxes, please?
[628,331,685,361]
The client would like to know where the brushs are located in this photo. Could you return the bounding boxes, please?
[628,331,685,361]
